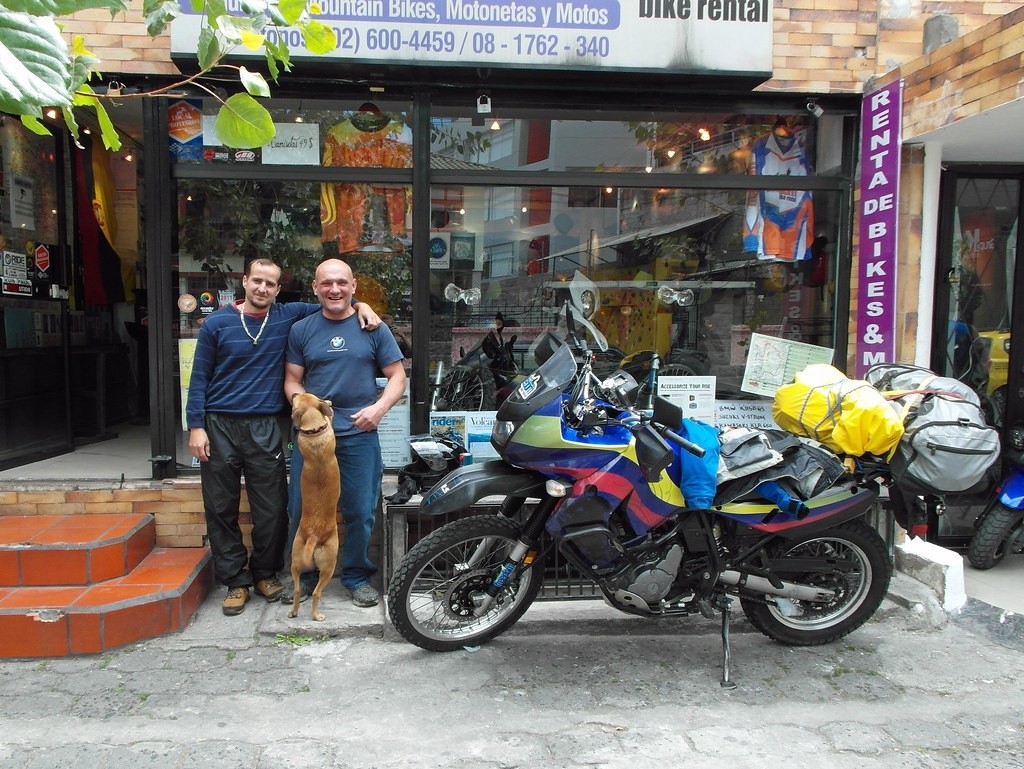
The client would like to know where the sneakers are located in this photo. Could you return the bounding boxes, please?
[223,577,284,616]
[284,576,379,607]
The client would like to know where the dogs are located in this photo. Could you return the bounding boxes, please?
[289,394,342,623]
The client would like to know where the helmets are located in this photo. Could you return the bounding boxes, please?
[398,434,469,493]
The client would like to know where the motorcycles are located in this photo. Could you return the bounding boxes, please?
[427,270,713,416]
[965,420,1024,572]
[384,295,900,692]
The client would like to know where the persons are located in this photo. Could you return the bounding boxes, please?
[188,258,383,615]
[280,261,408,606]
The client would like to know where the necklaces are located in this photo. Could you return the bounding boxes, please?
[238,303,271,346]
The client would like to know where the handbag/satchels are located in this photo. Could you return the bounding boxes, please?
[865,363,1003,498]
